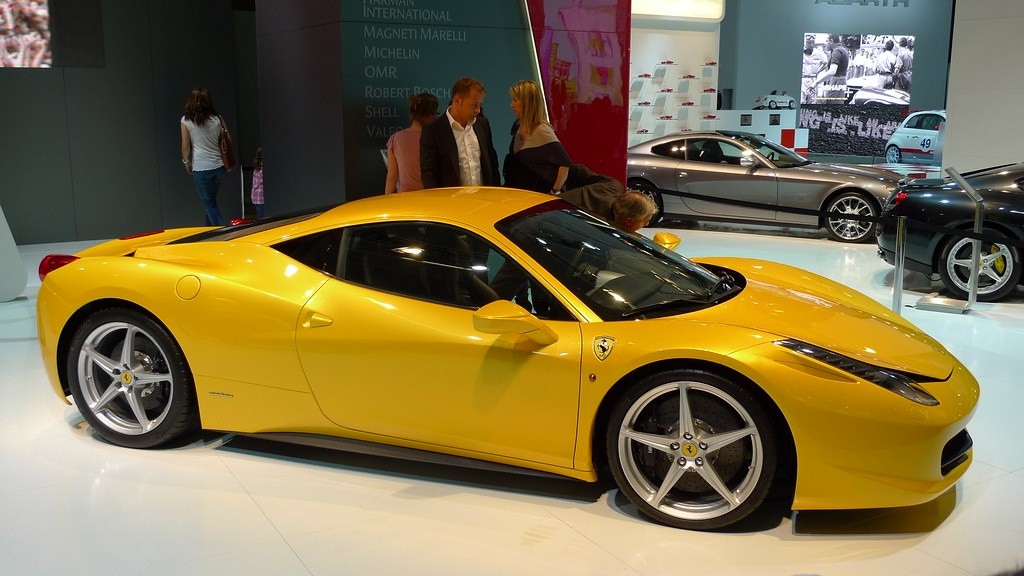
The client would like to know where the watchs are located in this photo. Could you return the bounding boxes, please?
[552,188,561,195]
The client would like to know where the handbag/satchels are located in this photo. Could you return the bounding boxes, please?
[218,129,237,171]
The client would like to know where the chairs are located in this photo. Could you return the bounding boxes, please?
[700,141,724,162]
[359,230,431,297]
[425,225,486,294]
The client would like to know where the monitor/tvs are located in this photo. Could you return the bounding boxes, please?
[0,0,56,73]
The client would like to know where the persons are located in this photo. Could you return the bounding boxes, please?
[740,142,762,166]
[181,87,232,227]
[560,163,659,231]
[806,33,849,104]
[384,93,440,195]
[801,33,828,104]
[847,49,875,79]
[251,147,265,218]
[503,80,571,314]
[420,77,501,284]
[873,38,913,92]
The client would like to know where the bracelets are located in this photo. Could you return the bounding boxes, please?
[183,158,189,163]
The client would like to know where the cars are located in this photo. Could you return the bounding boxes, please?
[883,109,948,163]
[847,86,910,107]
[753,90,796,110]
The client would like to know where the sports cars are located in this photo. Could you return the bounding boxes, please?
[874,162,1024,303]
[625,129,915,244]
[33,186,981,531]
